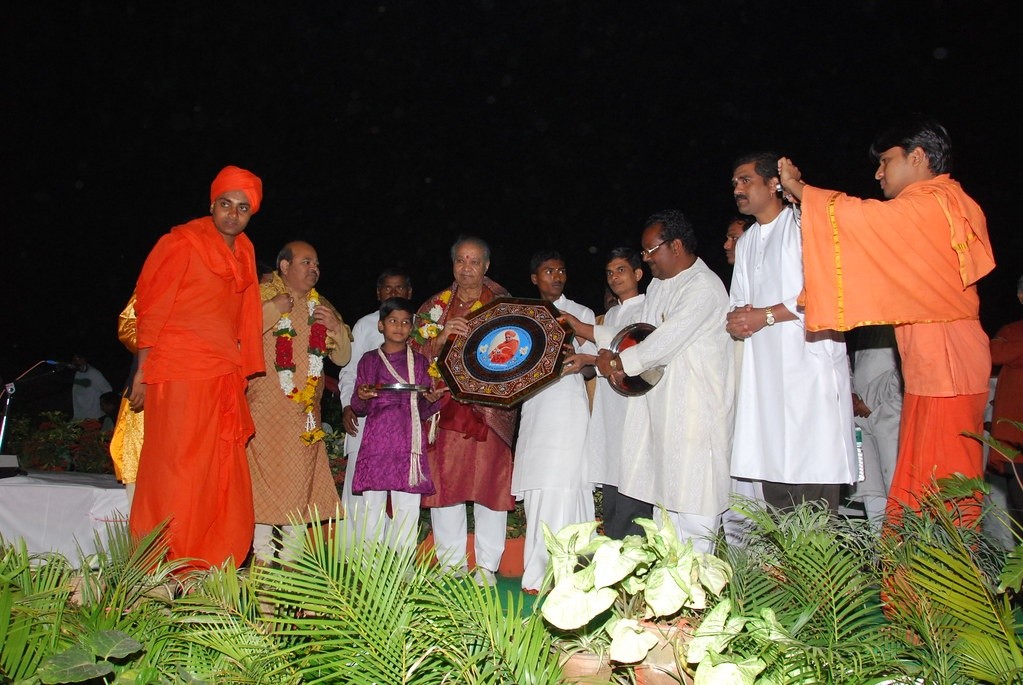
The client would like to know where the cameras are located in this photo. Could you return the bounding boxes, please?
[776,182,785,192]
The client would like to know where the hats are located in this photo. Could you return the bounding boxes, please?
[211,165,263,215]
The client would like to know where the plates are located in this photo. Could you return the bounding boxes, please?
[363,383,429,392]
[606,323,664,396]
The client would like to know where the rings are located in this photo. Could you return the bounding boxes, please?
[291,298,293,302]
[451,324,453,328]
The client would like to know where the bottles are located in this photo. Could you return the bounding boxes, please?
[854,422,865,482]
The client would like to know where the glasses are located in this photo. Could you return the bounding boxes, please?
[640,239,685,257]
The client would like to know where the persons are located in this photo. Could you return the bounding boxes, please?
[71,350,121,427]
[723,151,859,552]
[490,331,519,363]
[556,209,735,556]
[110,287,145,483]
[351,297,444,581]
[339,269,415,560]
[244,241,354,571]
[511,245,596,595]
[990,276,1023,518]
[128,166,266,583]
[411,235,511,586]
[778,122,996,646]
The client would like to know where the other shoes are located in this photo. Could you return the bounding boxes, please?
[280,561,301,577]
[241,559,272,575]
[429,565,468,579]
[522,576,547,593]
[473,568,498,588]
[400,565,418,583]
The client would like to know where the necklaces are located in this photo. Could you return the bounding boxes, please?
[273,287,327,446]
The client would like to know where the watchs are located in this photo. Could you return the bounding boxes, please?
[609,355,619,371]
[765,306,775,326]
